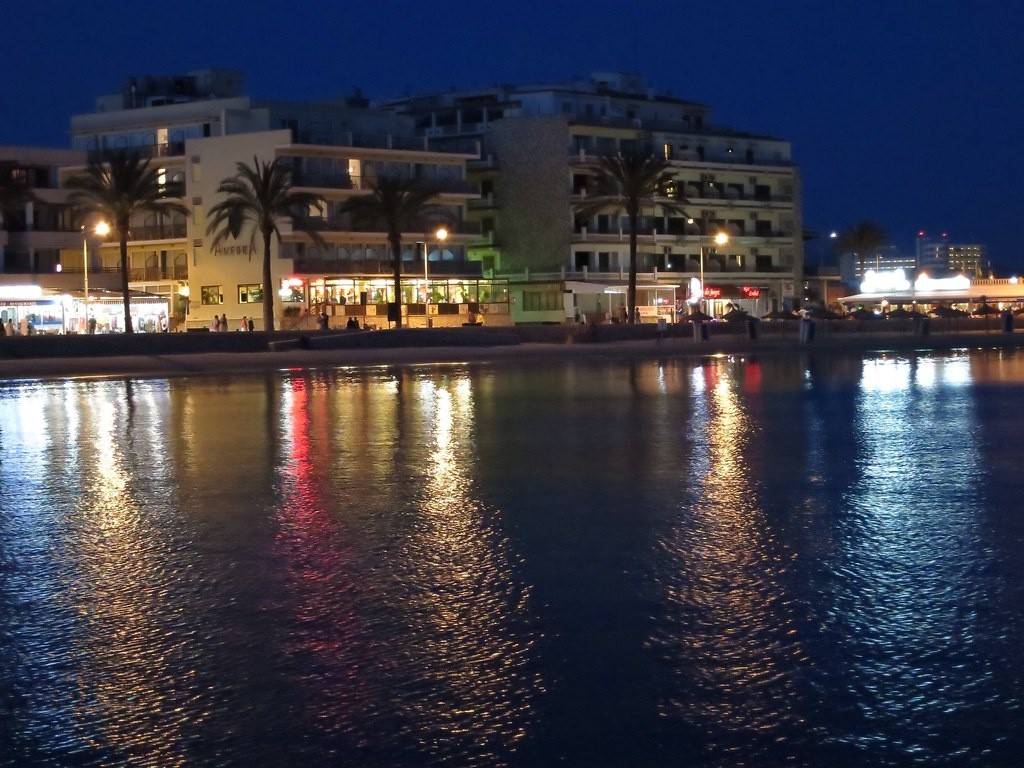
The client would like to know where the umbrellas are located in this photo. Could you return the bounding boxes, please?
[680,302,1024,344]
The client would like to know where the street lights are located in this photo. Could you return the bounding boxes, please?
[423,229,449,327]
[81,222,112,335]
[179,283,190,331]
[700,232,729,314]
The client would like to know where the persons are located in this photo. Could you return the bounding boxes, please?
[220,313,228,332]
[212,315,220,332]
[248,317,255,331]
[88,314,97,335]
[620,307,628,324]
[0,312,37,337]
[240,315,247,331]
[574,310,587,326]
[161,315,168,333]
[635,307,642,324]
[317,292,360,329]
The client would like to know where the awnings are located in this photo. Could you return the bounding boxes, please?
[0,298,65,308]
[68,290,169,305]
